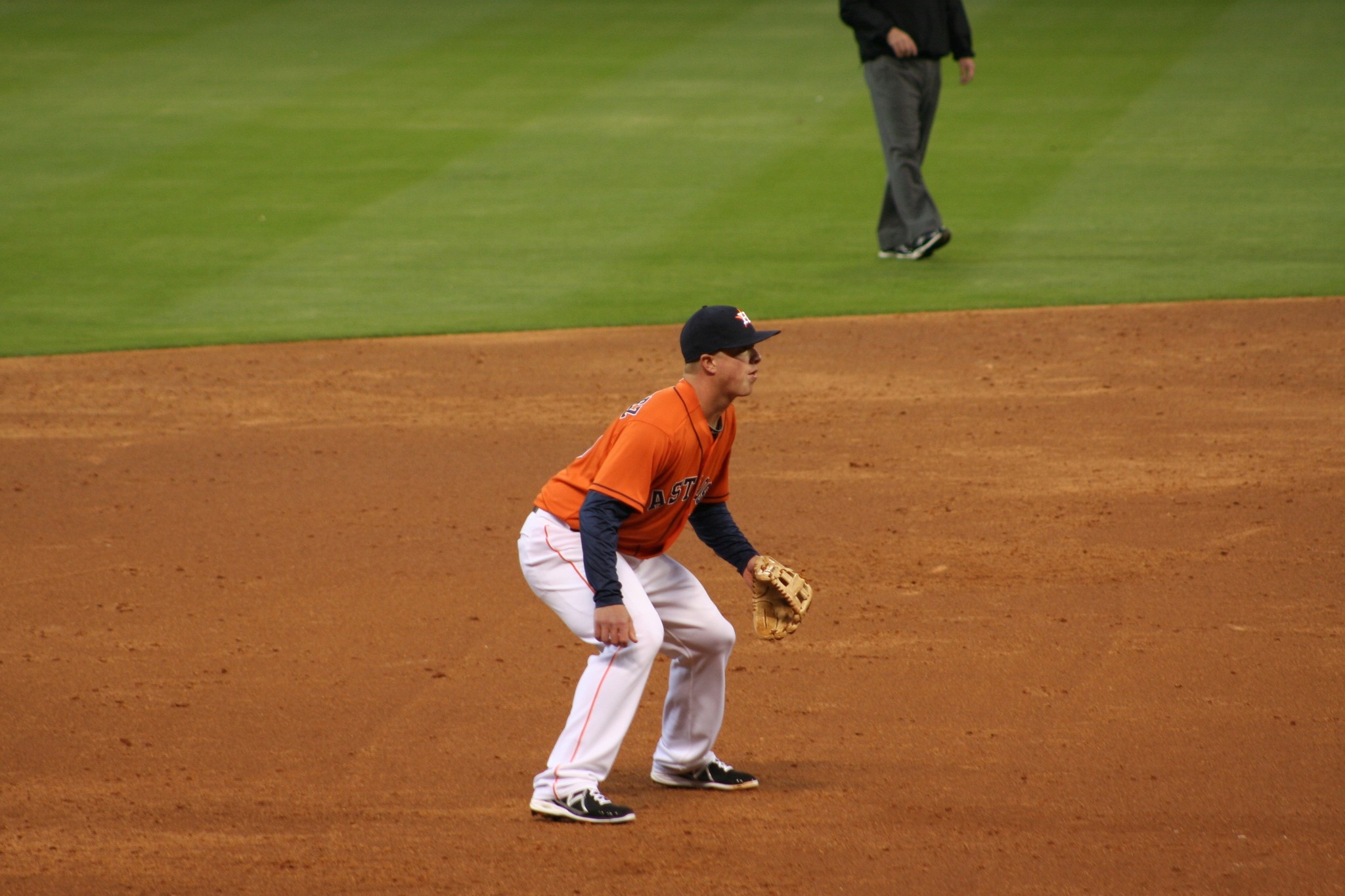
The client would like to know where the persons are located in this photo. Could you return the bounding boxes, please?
[835,0,978,261]
[517,304,811,825]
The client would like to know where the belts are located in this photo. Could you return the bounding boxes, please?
[532,506,580,532]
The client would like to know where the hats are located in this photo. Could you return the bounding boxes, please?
[680,305,781,363]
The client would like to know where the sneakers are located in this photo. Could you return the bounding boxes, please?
[529,788,635,824]
[912,227,950,260]
[878,242,913,259]
[650,756,758,791]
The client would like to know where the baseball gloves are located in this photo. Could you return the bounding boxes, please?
[752,555,813,644]
